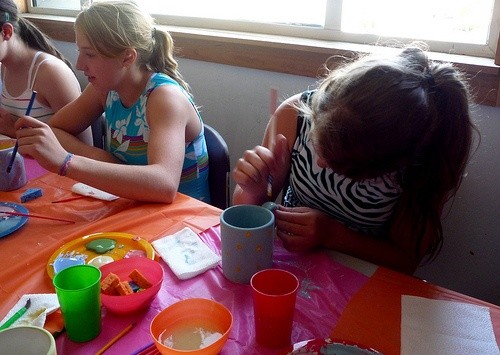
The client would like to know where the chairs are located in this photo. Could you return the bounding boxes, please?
[205,123,231,210]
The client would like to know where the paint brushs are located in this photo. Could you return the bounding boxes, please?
[0,211,76,224]
[131,342,155,355]
[52,195,84,203]
[0,298,32,331]
[267,87,279,197]
[95,322,139,355]
[7,90,38,173]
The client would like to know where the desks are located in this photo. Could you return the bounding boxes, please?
[0,135,500,355]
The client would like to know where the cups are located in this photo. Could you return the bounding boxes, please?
[0,138,27,191]
[250,269,299,348]
[220,200,280,284]
[52,264,103,342]
[0,325,57,355]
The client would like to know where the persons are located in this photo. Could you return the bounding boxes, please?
[14,6,209,204]
[0,0,94,148]
[234,46,474,272]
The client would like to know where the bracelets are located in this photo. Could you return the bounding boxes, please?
[58,153,74,176]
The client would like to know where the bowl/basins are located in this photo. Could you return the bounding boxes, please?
[99,257,164,317]
[150,297,233,355]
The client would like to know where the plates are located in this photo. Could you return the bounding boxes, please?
[287,338,383,355]
[46,231,154,280]
[0,201,29,237]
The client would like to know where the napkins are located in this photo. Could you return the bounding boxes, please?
[71,182,119,201]
[151,227,221,280]
[0,293,60,333]
[401,296,500,355]
